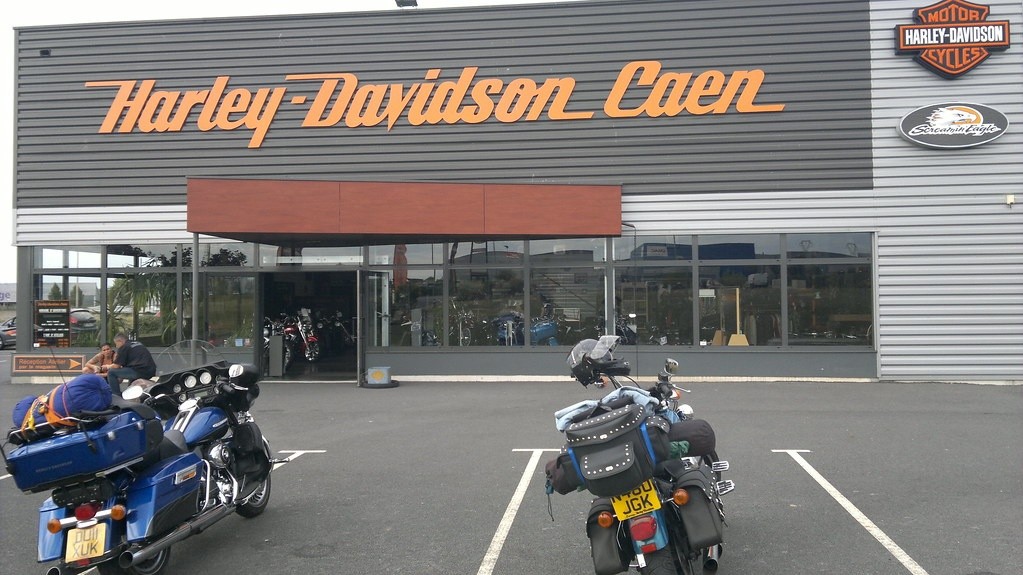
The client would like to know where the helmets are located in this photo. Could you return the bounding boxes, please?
[566,339,612,387]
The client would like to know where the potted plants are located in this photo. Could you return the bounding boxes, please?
[233,312,253,347]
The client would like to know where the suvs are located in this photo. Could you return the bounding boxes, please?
[0,308,97,348]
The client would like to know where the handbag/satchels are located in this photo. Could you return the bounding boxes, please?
[565,403,656,499]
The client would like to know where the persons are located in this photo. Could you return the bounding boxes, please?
[83,343,123,383]
[102,333,157,395]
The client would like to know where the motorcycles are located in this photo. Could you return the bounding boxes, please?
[401,292,635,346]
[552,332,736,575]
[215,304,353,378]
[0,338,291,575]
